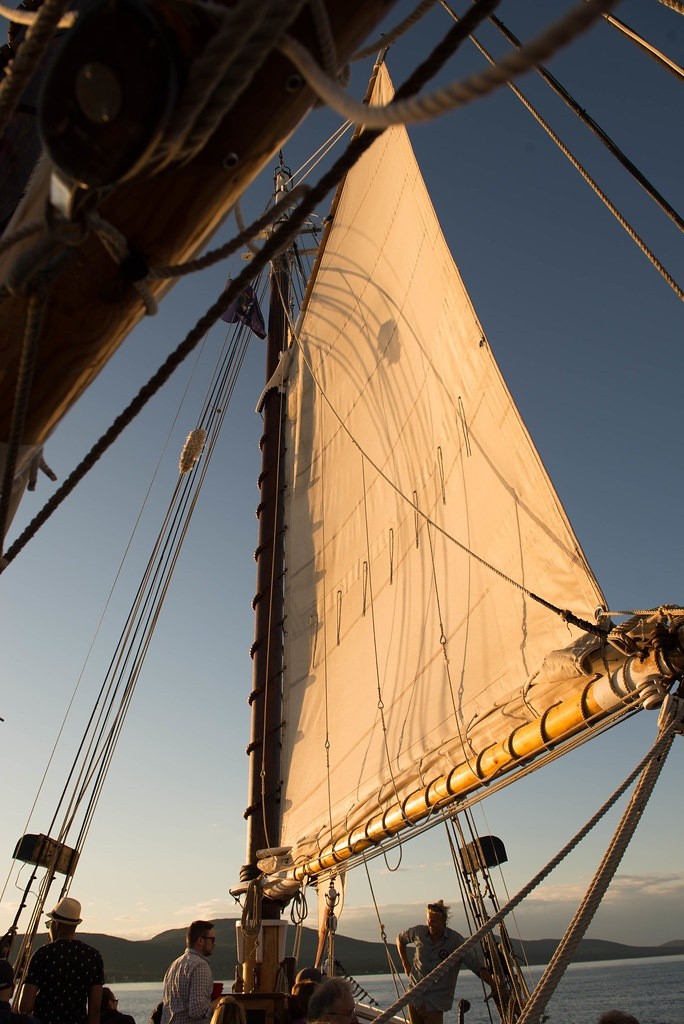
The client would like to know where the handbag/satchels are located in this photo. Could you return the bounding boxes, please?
[150,1002,163,1024]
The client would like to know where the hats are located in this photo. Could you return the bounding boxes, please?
[0,960,14,989]
[46,897,82,925]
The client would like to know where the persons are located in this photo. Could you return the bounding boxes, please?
[276,957,357,1024]
[597,1010,640,1024]
[162,920,222,1024]
[150,1001,166,1024]
[20,898,105,1024]
[396,900,501,1024]
[102,987,135,1024]
[0,959,21,1024]
[210,997,247,1024]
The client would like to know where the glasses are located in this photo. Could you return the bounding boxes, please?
[326,1002,356,1018]
[427,904,445,918]
[112,997,118,1005]
[45,919,52,929]
[202,936,215,944]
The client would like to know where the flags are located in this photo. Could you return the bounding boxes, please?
[218,279,267,340]
